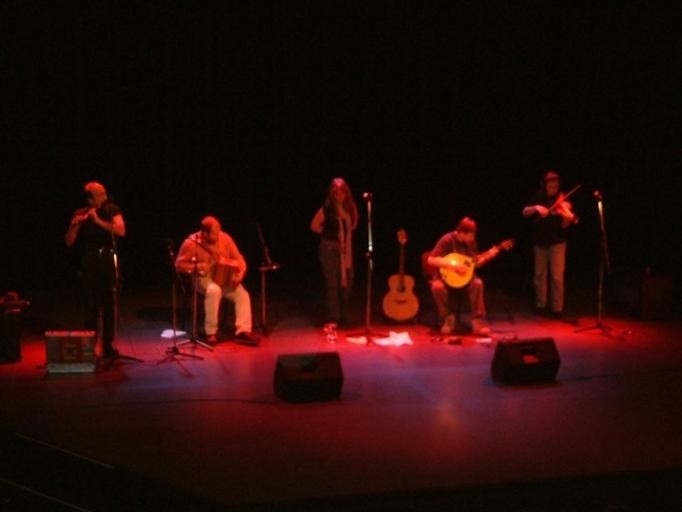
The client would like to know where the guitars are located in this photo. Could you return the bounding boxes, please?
[381,229,420,322]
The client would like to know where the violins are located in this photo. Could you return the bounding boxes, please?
[552,193,577,223]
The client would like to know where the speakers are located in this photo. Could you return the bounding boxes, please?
[273,352,345,404]
[490,337,561,386]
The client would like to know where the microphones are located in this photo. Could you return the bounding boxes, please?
[362,192,372,200]
[594,190,602,200]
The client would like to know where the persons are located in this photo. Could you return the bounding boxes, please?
[174,214,259,350]
[522,168,575,321]
[66,178,128,360]
[310,176,362,334]
[427,213,499,336]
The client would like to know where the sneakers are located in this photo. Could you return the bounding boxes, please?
[533,305,562,323]
[441,314,456,334]
[235,332,256,345]
[472,319,490,335]
[207,335,217,343]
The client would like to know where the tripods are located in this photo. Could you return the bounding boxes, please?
[157,254,204,377]
[346,191,390,346]
[574,192,613,341]
[167,233,215,353]
[99,223,144,372]
